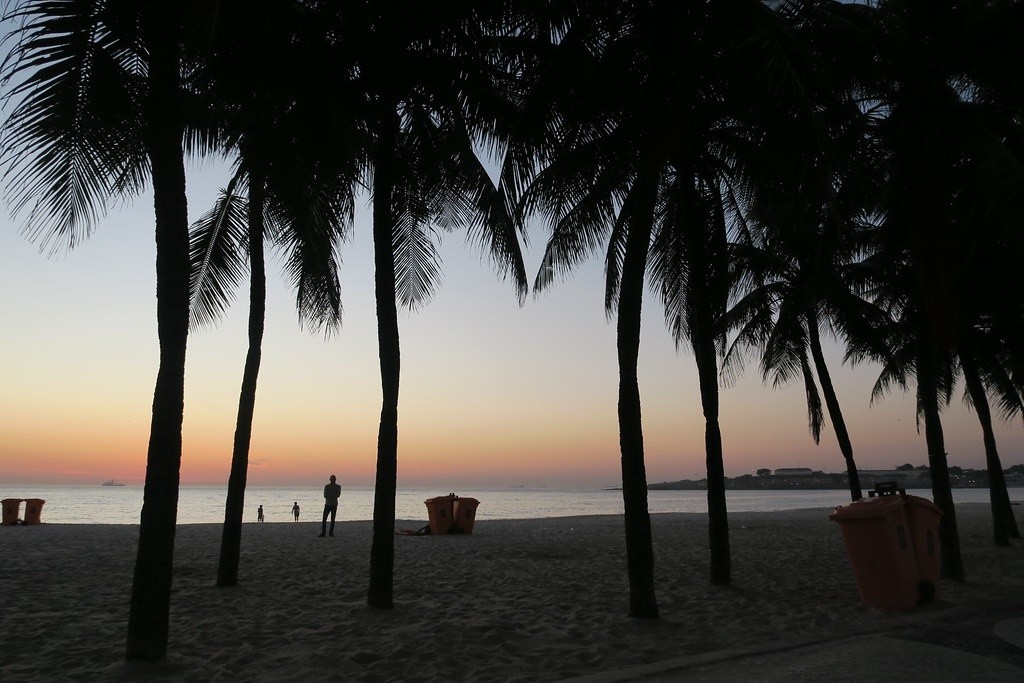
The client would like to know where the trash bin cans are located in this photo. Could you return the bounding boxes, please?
[423,492,480,537]
[24,498,47,525]
[2,498,24,525]
[827,479,948,615]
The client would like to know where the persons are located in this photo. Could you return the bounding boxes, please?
[292,502,300,522]
[318,474,341,537]
[258,505,265,523]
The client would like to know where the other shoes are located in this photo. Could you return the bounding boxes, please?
[329,534,334,537]
[318,533,325,537]
